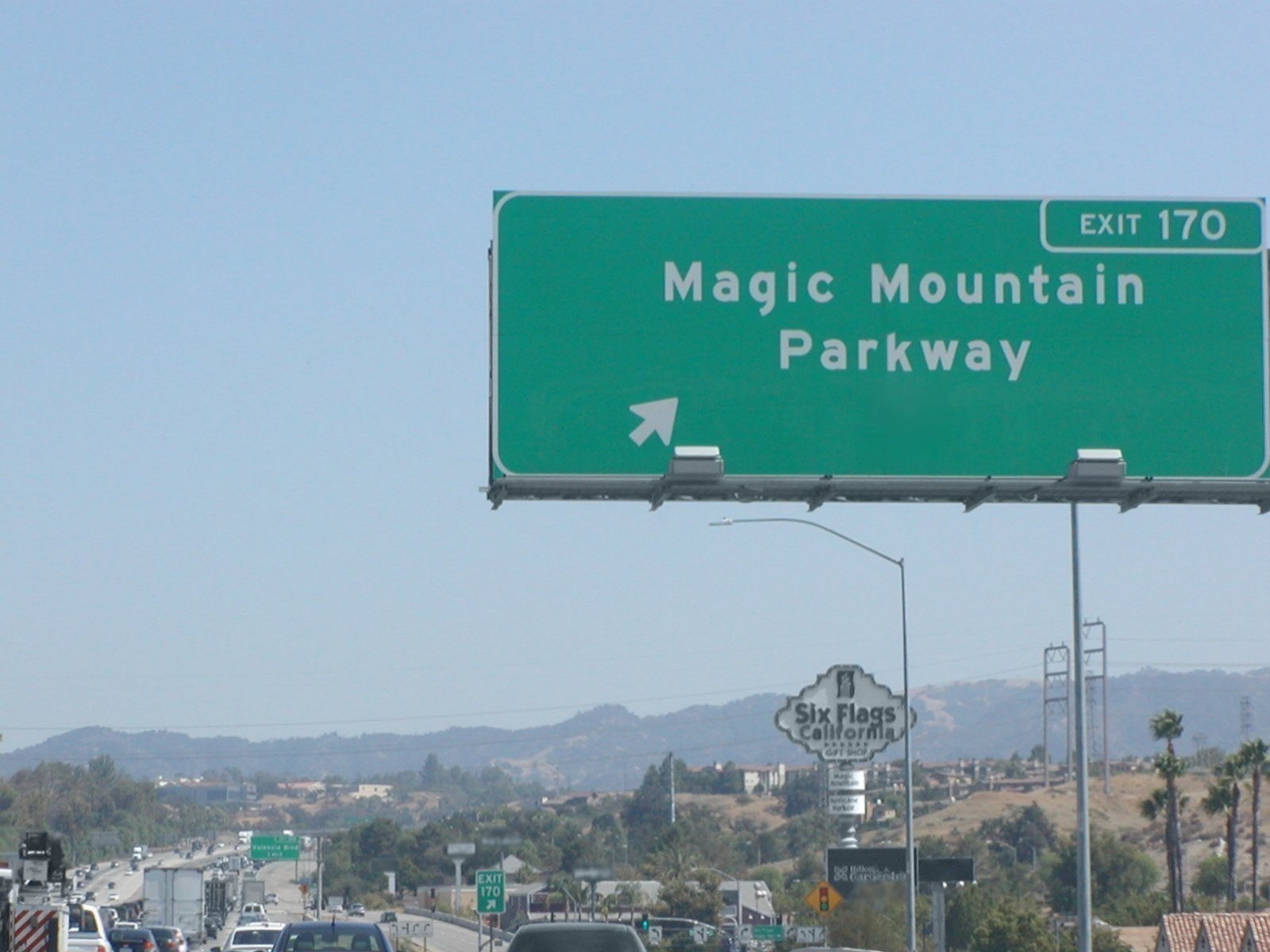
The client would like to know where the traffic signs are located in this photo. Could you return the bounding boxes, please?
[475,868,505,916]
[751,925,785,942]
[479,188,1266,483]
[252,834,302,861]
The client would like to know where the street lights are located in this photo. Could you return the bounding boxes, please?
[686,866,744,952]
[704,518,919,952]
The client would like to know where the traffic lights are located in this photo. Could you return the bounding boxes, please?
[642,911,650,933]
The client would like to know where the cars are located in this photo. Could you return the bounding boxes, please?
[305,899,314,910]
[211,925,285,952]
[380,912,397,923]
[60,836,265,952]
[264,894,279,903]
[270,917,394,952]
[347,903,366,917]
[503,921,649,952]
[327,903,343,913]
